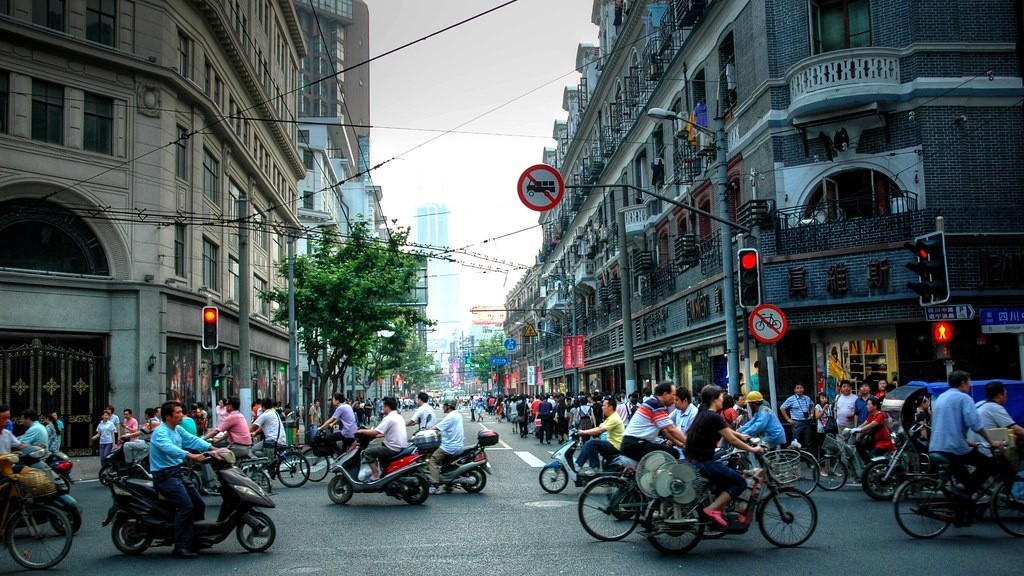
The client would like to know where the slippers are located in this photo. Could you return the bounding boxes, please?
[703,508,728,526]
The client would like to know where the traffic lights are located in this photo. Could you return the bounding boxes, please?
[202,306,219,349]
[737,249,761,308]
[904,229,954,345]
[211,363,226,388]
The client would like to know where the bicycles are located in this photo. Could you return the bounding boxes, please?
[889,432,1024,543]
[300,426,378,483]
[0,469,73,570]
[577,434,823,556]
[232,436,311,487]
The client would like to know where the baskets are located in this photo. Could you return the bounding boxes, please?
[16,468,57,499]
[991,442,1024,471]
[763,449,801,484]
[822,433,837,450]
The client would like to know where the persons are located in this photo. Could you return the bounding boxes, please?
[0,371,1023,556]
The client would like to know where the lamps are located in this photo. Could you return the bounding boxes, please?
[145,274,155,283]
[145,56,157,63]
[149,352,156,368]
[165,277,175,284]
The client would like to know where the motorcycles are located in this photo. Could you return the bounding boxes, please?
[858,379,1024,501]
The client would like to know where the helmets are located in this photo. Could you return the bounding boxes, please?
[745,391,763,403]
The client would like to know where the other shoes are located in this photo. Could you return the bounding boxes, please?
[954,523,972,528]
[201,543,213,549]
[820,472,828,476]
[171,548,197,559]
[951,486,971,502]
[871,485,881,491]
[834,473,843,476]
[809,464,814,469]
[363,478,379,483]
[204,488,221,495]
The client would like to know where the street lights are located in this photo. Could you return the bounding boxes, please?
[287,221,337,428]
[647,107,743,399]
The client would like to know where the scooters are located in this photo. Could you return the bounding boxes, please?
[97,433,276,557]
[812,427,899,492]
[325,425,441,505]
[607,432,745,521]
[408,414,500,493]
[537,408,639,494]
[180,437,280,499]
[0,443,83,538]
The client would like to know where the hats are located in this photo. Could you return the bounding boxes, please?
[444,397,457,407]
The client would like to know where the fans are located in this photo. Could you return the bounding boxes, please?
[636,450,704,506]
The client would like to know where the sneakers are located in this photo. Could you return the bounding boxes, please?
[429,487,442,494]
[584,467,600,476]
[443,486,454,491]
[573,463,582,473]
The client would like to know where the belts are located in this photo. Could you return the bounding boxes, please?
[234,442,250,446]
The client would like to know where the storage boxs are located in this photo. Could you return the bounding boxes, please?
[413,429,443,449]
[477,429,499,447]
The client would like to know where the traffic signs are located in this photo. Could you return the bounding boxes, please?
[926,303,976,320]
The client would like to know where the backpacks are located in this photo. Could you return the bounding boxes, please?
[818,406,839,436]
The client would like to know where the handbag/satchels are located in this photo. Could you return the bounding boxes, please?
[312,436,335,457]
[262,441,277,458]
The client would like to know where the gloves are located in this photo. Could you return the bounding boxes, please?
[850,428,863,434]
